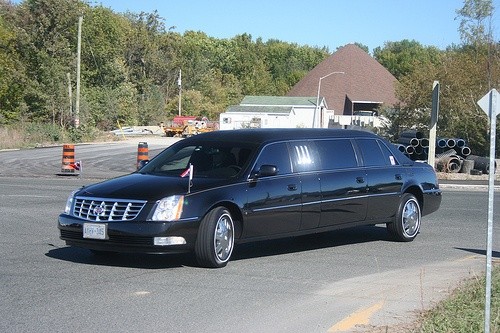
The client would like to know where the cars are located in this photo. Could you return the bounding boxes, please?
[57,128,442,269]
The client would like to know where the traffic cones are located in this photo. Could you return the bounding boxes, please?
[56,145,79,176]
[138,142,149,160]
